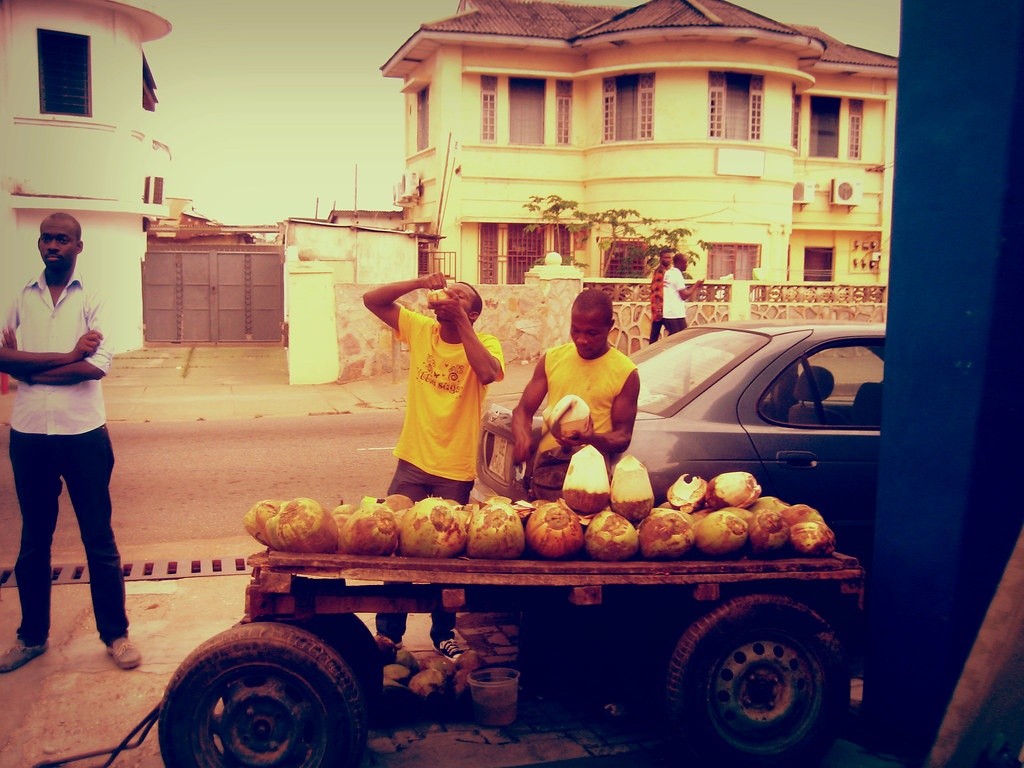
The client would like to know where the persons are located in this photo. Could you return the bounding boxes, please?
[363,273,506,663]
[0,212,140,673]
[510,290,641,468]
[663,253,704,335]
[649,250,675,343]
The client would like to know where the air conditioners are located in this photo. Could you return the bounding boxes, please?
[401,169,421,198]
[792,181,815,205]
[393,183,409,203]
[830,178,860,206]
[144,176,165,204]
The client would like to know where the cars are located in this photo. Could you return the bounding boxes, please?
[469,319,886,559]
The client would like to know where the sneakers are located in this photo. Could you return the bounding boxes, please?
[106,635,141,670]
[0,640,49,672]
[373,634,403,649]
[432,638,462,663]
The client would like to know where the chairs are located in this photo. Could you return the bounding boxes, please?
[847,379,880,429]
[789,366,845,430]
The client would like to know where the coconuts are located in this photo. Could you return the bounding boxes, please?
[547,395,590,439]
[372,632,487,698]
[242,444,837,562]
